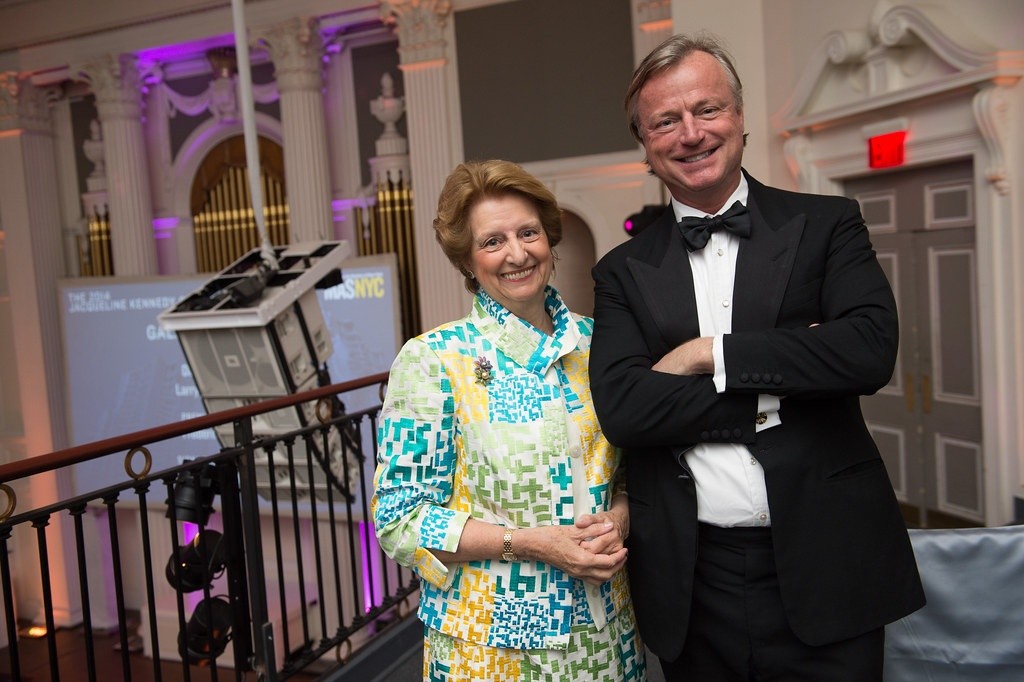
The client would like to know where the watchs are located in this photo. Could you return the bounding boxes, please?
[502,526,520,564]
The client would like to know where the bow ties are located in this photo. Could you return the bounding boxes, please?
[679,200,752,253]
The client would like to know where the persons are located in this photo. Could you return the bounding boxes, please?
[372,159,650,682]
[587,33,928,681]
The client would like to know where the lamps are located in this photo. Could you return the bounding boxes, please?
[165,456,220,525]
[165,529,226,594]
[177,595,231,667]
[226,271,275,305]
